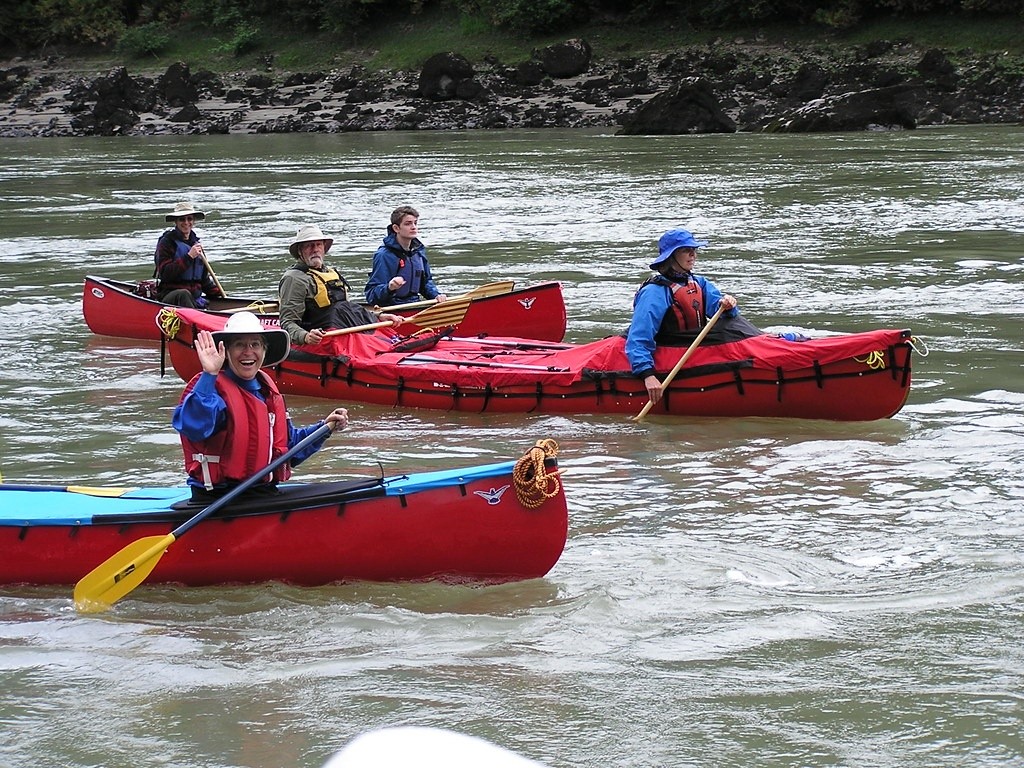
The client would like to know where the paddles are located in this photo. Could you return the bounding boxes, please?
[321,296,474,338]
[632,301,727,422]
[373,280,515,313]
[199,247,227,297]
[74,419,339,609]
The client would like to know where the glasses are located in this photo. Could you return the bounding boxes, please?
[177,217,194,223]
[229,341,263,351]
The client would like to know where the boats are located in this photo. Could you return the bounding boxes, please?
[163,304,914,423]
[0,440,571,585]
[82,275,567,345]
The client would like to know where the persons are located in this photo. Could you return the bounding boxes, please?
[154,207,223,309]
[278,236,405,356]
[365,207,449,305]
[174,314,351,500]
[626,229,748,407]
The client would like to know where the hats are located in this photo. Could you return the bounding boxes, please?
[208,311,289,368]
[289,224,333,258]
[166,202,205,221]
[649,228,707,269]
